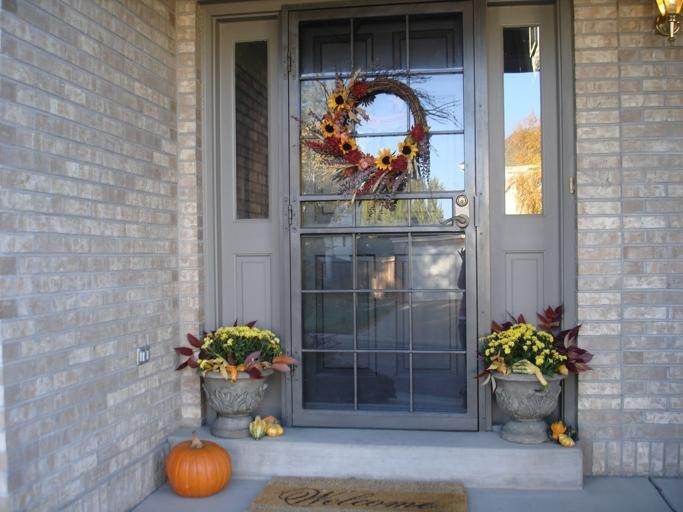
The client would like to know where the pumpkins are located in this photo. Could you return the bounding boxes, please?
[250,415,284,440]
[550,420,576,447]
[164,431,232,498]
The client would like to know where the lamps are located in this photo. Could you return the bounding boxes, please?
[655,0,683,40]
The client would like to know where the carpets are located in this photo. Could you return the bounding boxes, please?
[251,475,467,512]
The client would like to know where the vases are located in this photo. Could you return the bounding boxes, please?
[489,371,568,444]
[197,367,275,439]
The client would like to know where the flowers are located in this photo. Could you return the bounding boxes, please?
[174,316,299,382]
[473,302,594,386]
[299,67,431,211]
